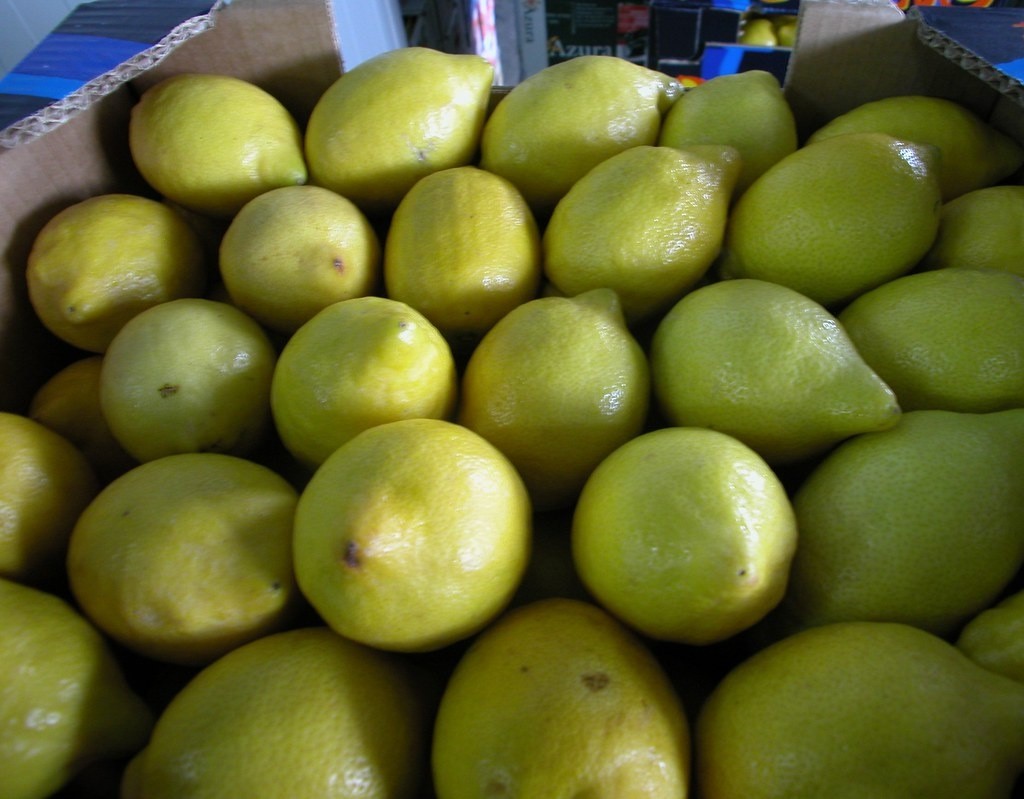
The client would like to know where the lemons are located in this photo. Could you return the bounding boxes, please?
[0,48,1024,799]
